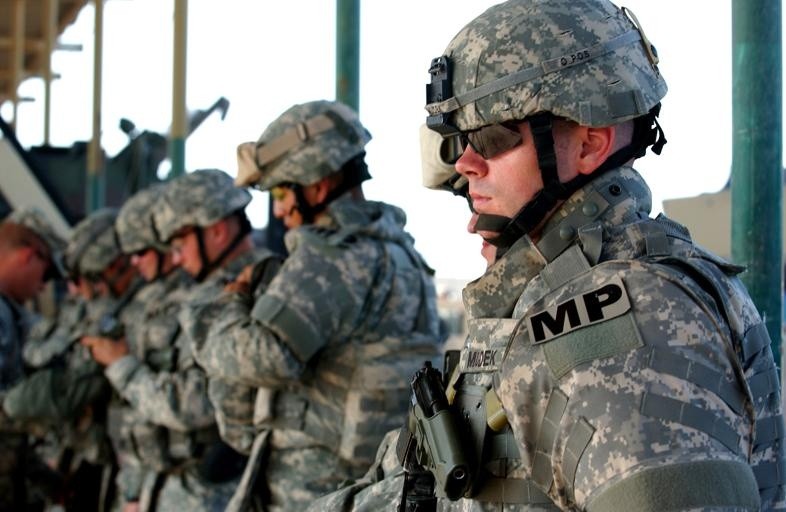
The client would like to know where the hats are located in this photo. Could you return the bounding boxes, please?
[3,201,70,254]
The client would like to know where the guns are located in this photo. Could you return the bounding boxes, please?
[396,362,471,502]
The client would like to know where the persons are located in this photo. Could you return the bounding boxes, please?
[306,0,785,512]
[412,123,496,272]
[2,99,443,512]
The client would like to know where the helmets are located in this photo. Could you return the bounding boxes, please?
[61,167,256,280]
[416,119,472,194]
[248,98,375,192]
[424,0,670,140]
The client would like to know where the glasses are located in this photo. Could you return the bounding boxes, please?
[66,246,151,288]
[435,112,582,166]
[270,184,290,201]
[18,237,60,285]
